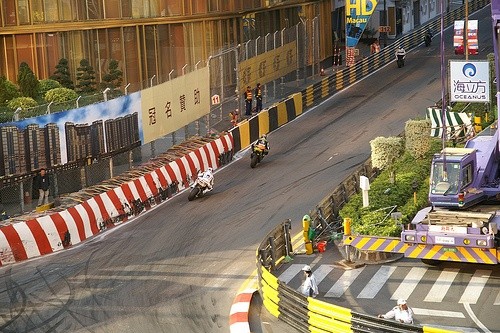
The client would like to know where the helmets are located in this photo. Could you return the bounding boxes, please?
[207,167,213,174]
[260,134,267,140]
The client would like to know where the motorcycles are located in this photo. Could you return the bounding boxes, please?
[251,144,269,168]
[396,54,405,68]
[186,175,214,201]
[424,35,433,47]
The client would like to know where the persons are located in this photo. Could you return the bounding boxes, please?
[378,298,415,325]
[424,29,433,42]
[301,264,319,298]
[369,40,380,56]
[395,45,406,61]
[252,134,270,156]
[255,83,263,112]
[36,168,50,207]
[191,167,215,198]
[243,86,253,116]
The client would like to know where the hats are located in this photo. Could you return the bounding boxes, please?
[302,266,312,272]
[397,299,407,305]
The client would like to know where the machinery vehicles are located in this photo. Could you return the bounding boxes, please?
[427,127,500,212]
[343,211,500,267]
